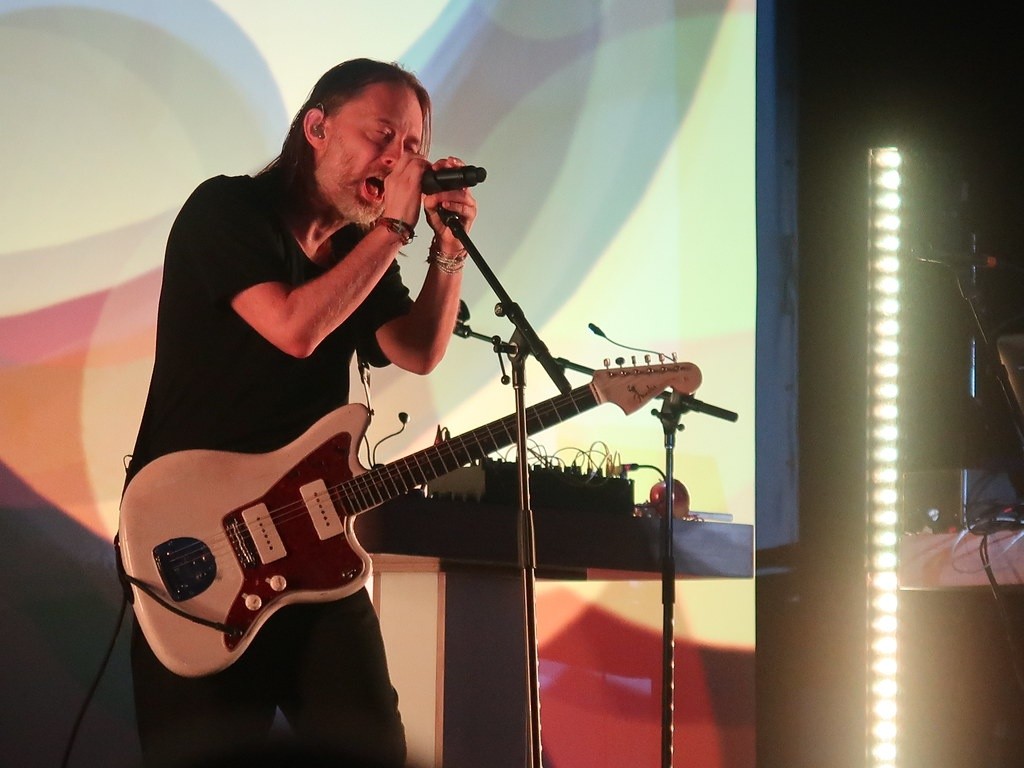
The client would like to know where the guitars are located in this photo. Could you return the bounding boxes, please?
[116,352,703,680]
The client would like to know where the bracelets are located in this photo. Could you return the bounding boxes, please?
[377,217,413,245]
[427,245,466,273]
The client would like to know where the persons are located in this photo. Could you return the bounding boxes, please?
[113,59,478,768]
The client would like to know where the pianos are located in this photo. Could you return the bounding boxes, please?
[349,489,755,583]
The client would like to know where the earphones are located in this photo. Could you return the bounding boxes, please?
[311,124,326,139]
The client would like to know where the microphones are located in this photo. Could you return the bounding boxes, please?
[420,167,487,195]
[909,243,1014,270]
